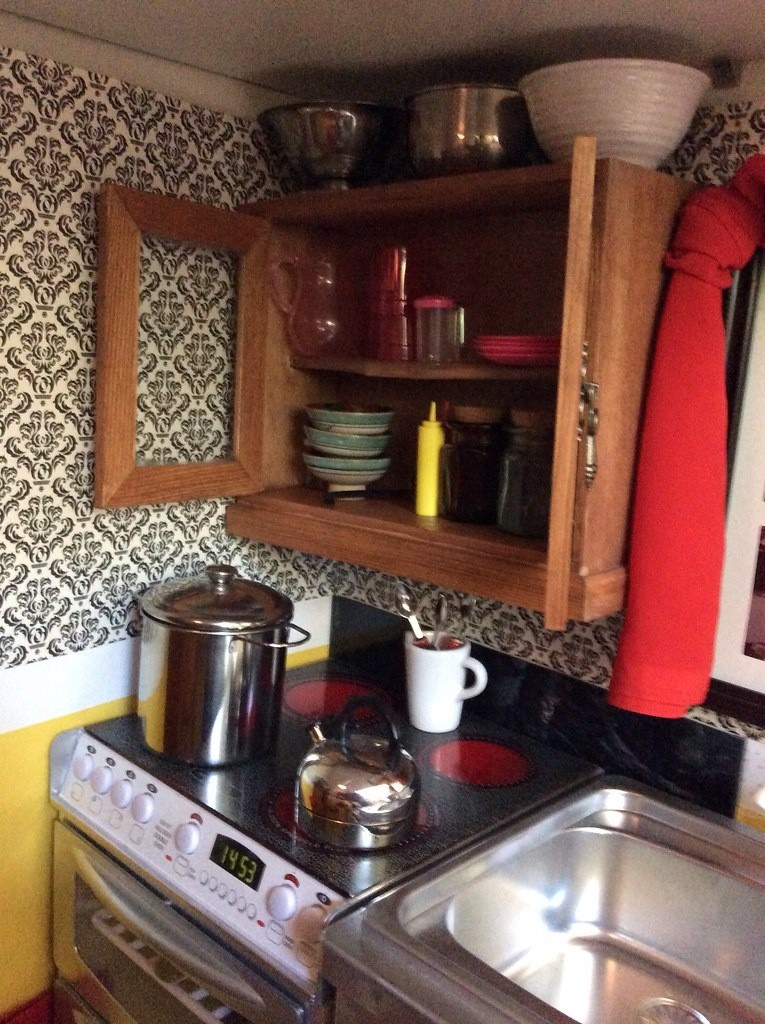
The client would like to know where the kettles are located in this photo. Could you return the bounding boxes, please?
[296,692,421,849]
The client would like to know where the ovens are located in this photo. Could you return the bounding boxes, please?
[44,816,332,1022]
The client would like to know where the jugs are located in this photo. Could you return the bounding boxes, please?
[268,232,360,359]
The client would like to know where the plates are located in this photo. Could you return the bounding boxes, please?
[470,333,560,367]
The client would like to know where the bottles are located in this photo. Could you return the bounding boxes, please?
[416,401,447,517]
[362,243,429,362]
[413,294,464,364]
[443,404,510,524]
[494,405,556,537]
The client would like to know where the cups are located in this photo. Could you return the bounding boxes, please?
[405,633,488,735]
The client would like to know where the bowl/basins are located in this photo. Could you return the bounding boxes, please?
[518,57,711,172]
[255,99,400,195]
[304,403,397,435]
[299,424,389,457]
[402,84,531,172]
[300,452,390,502]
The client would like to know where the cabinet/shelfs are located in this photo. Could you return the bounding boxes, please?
[93,133,700,631]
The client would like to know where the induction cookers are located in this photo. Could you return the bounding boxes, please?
[49,649,606,1002]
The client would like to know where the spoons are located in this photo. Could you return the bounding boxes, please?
[393,581,430,648]
[451,594,484,651]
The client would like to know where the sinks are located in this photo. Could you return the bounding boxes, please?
[360,775,764,1023]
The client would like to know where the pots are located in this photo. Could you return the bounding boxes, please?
[428,592,449,650]
[130,562,313,769]
